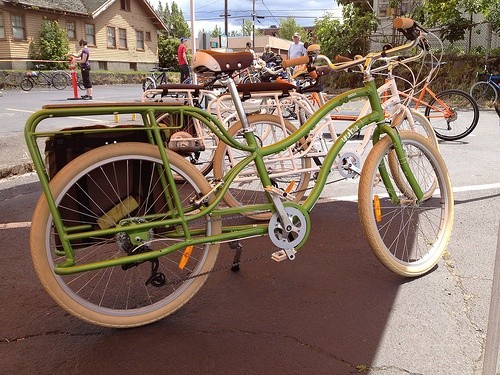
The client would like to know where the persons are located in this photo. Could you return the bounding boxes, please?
[243,41,258,67]
[70,38,93,100]
[287,33,307,78]
[261,44,275,62]
[178,37,190,85]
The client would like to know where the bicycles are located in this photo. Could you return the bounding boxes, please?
[469,63,500,109]
[20,65,88,92]
[143,58,481,184]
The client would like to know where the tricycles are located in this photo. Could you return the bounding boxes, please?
[22,16,454,329]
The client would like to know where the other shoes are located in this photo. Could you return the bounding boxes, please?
[81,95,88,97]
[84,96,92,100]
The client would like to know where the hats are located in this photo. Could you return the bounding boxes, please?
[180,38,187,42]
[294,33,299,37]
[246,42,252,46]
